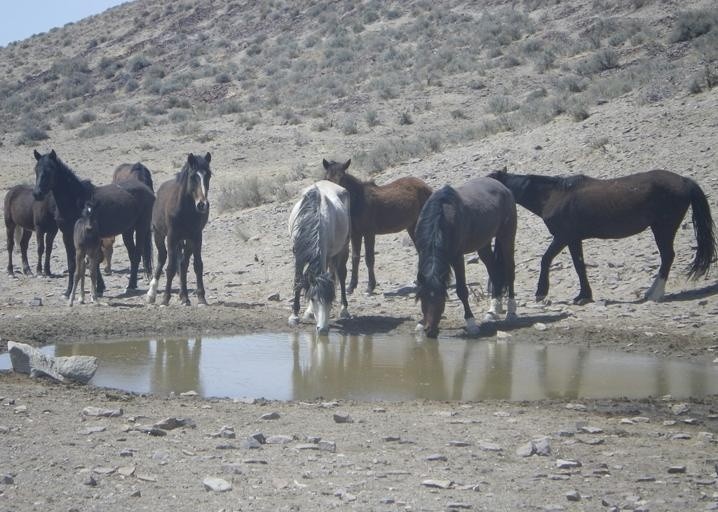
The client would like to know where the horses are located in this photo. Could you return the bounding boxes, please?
[145,152,215,310]
[322,157,433,299]
[4,149,155,309]
[486,165,718,303]
[413,176,519,339]
[287,180,351,337]
